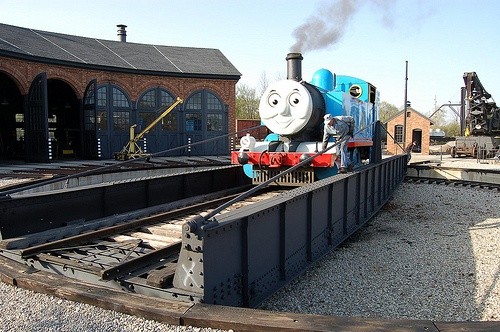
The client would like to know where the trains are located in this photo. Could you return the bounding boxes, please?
[229,51,381,188]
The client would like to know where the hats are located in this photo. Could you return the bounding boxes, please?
[323,114,333,124]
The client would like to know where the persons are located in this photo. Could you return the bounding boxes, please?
[405,141,417,162]
[321,114,355,173]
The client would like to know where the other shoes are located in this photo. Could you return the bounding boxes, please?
[337,168,347,174]
[347,163,354,171]
